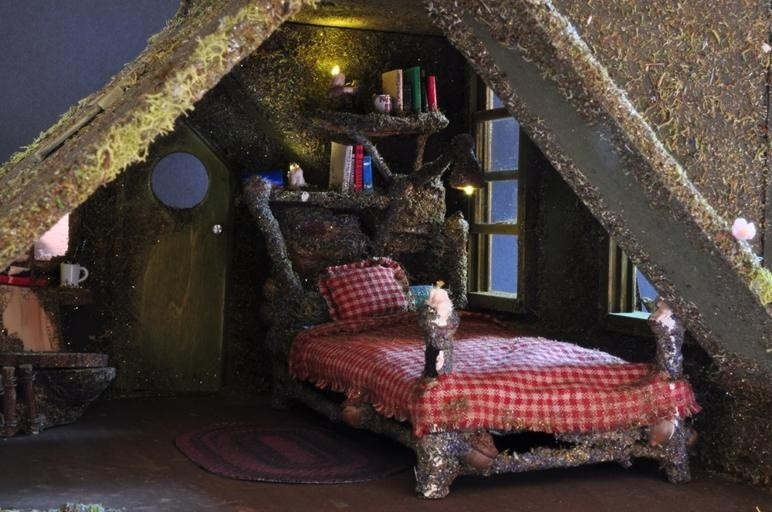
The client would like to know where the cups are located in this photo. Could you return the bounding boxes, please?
[373,94,392,115]
[60,263,88,289]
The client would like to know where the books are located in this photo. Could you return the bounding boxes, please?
[1,272,52,290]
[328,67,441,194]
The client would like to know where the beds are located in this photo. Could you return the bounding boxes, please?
[246,172,703,499]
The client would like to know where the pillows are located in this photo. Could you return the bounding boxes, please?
[317,254,415,319]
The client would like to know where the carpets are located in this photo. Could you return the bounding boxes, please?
[174,419,416,486]
